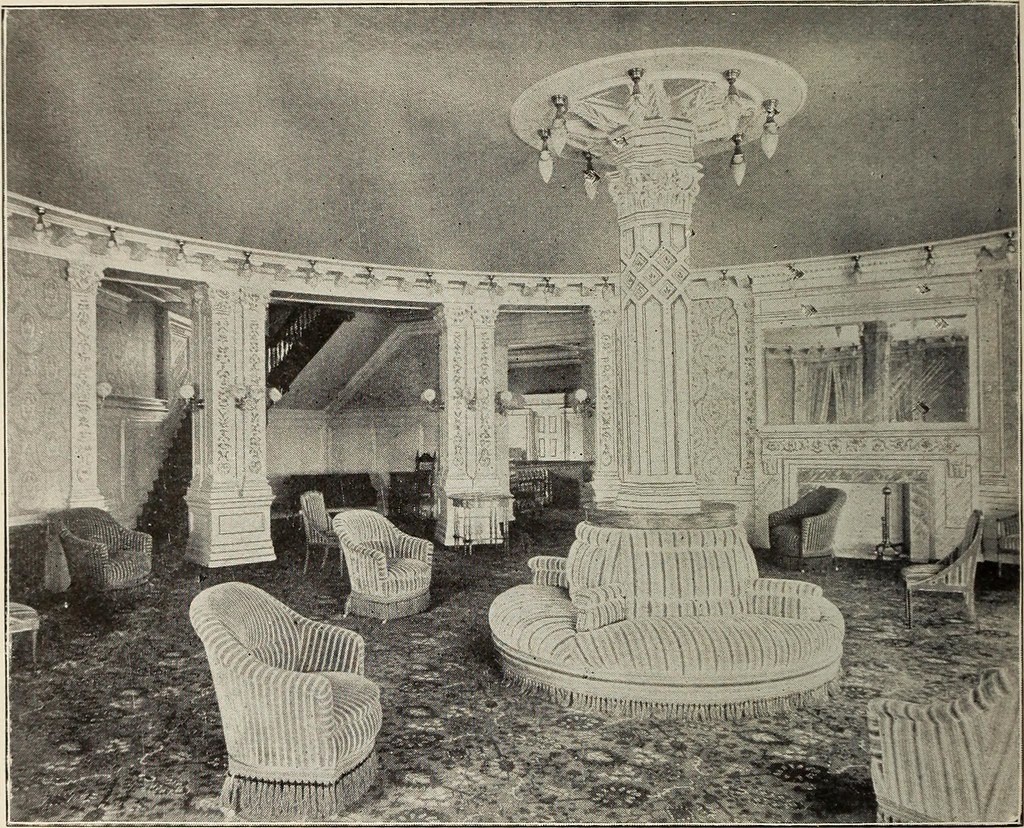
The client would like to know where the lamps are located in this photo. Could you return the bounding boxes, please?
[421,389,445,412]
[511,48,806,201]
[96,381,112,408]
[577,390,596,414]
[495,389,512,415]
[267,389,282,410]
[180,387,202,412]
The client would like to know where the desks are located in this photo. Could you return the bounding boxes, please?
[450,492,514,555]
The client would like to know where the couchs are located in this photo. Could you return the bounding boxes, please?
[867,667,1020,823]
[489,521,845,718]
[768,487,846,570]
[333,509,433,620]
[269,474,377,516]
[48,508,153,599]
[190,581,384,816]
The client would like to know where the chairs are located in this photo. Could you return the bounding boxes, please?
[300,489,345,579]
[995,513,1020,578]
[901,509,983,628]
[9,603,39,662]
